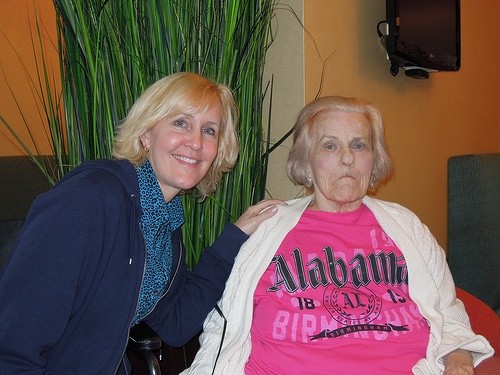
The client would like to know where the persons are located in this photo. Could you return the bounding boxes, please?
[177,96,494,374]
[0,70,288,375]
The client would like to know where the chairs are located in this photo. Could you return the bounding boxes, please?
[0,156,161,375]
[456,287,500,374]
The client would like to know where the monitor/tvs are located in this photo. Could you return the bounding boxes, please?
[386,0,461,71]
